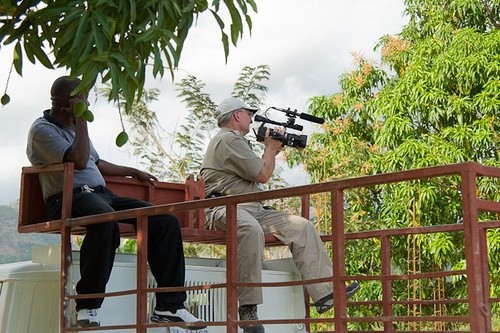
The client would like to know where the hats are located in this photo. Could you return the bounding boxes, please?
[213,96,259,118]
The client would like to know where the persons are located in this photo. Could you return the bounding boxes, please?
[199,97,360,333]
[26,75,208,330]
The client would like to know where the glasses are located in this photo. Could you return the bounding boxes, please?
[238,110,253,117]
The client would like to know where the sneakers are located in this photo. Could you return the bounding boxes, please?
[76,306,101,327]
[239,304,265,333]
[150,306,196,330]
[316,282,360,313]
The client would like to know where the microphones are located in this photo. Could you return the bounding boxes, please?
[272,107,324,124]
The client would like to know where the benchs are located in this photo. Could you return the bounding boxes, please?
[18,162,285,246]
